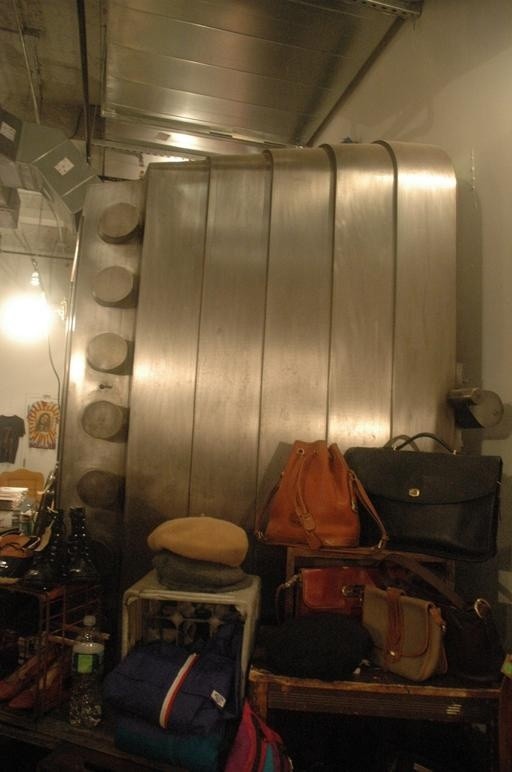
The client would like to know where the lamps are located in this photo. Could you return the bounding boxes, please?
[1,194,58,345]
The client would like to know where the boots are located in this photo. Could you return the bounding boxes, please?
[24,507,98,585]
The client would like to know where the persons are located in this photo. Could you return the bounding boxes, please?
[35,413,50,431]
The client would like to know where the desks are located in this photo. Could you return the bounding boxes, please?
[120,570,259,707]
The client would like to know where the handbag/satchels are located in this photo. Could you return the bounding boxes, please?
[254,432,501,557]
[103,640,293,772]
[252,566,501,681]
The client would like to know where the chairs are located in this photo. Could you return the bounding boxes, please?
[0,469,47,587]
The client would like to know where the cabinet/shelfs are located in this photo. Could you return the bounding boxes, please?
[250,536,511,770]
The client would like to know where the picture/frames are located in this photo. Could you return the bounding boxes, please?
[27,393,60,449]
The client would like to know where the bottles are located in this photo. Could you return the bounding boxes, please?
[19,497,32,538]
[66,614,104,729]
